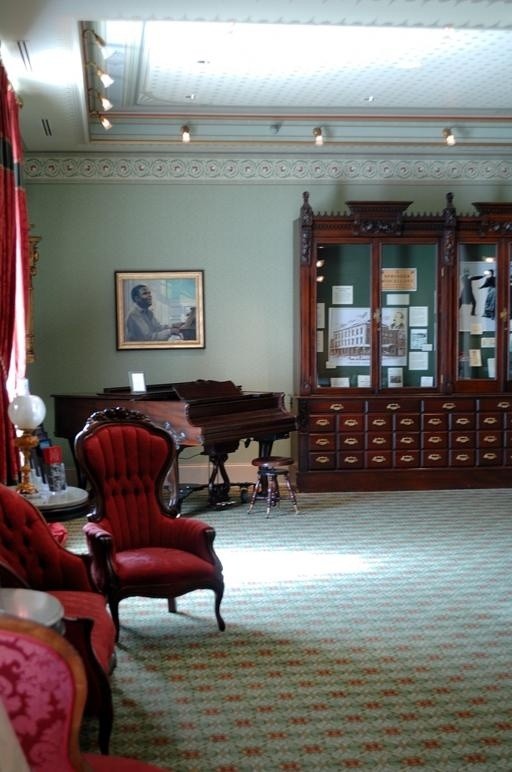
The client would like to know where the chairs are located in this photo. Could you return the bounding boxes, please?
[0,615,165,772]
[1,482,125,754]
[71,405,227,645]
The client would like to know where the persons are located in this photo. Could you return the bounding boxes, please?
[125,285,185,341]
[390,313,404,329]
[479,270,496,320]
[459,269,487,315]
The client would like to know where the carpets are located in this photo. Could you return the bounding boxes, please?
[53,484,512,771]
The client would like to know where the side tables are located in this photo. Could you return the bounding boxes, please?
[0,587,65,636]
[7,485,92,525]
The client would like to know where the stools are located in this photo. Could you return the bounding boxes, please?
[244,455,302,519]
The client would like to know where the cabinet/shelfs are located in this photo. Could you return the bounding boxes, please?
[289,188,512,494]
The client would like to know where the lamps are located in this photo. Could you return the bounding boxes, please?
[441,129,457,146]
[82,28,138,65]
[312,127,333,149]
[86,61,114,88]
[88,87,114,111]
[7,394,48,499]
[89,110,112,130]
[180,126,194,143]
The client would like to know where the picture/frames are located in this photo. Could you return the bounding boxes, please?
[114,270,208,351]
[128,369,148,395]
